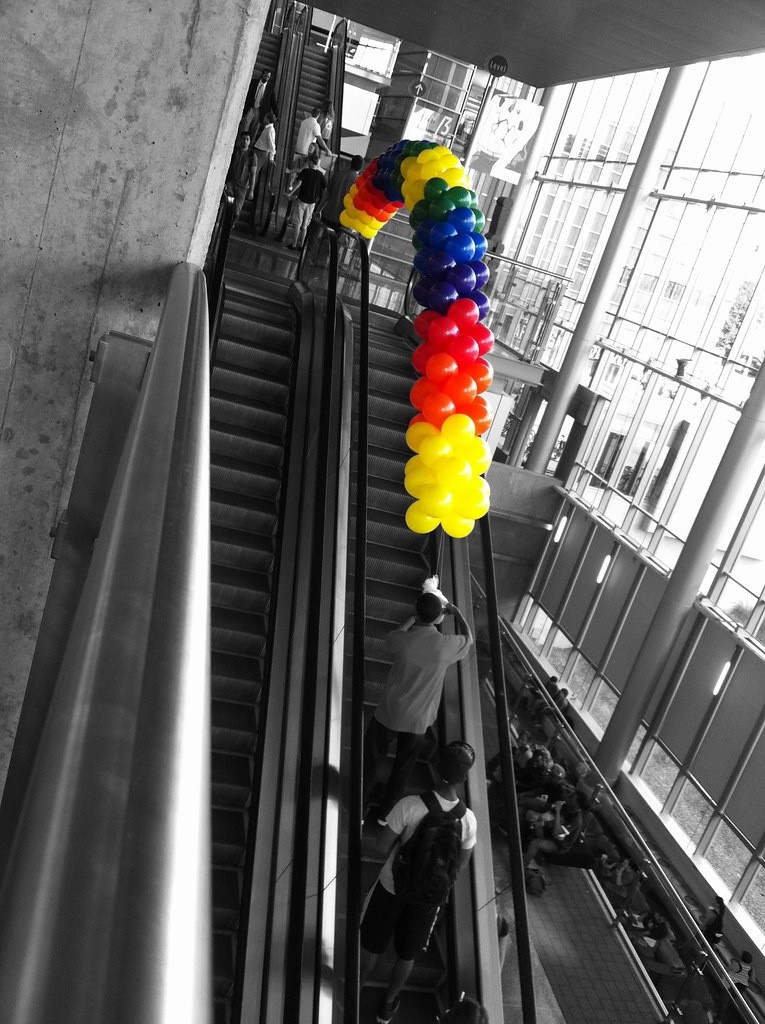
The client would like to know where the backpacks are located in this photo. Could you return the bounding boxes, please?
[393,791,467,907]
[710,909,723,944]
[321,116,333,141]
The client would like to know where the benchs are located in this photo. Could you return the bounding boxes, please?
[503,637,765,1024]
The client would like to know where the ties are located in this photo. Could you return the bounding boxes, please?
[255,84,263,101]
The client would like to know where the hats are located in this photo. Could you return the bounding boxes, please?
[547,764,566,779]
[437,741,475,769]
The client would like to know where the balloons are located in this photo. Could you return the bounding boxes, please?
[340,139,493,538]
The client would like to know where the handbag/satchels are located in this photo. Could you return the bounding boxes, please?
[525,867,546,897]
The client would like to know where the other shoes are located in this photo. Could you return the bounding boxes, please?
[289,186,293,190]
[377,992,400,1023]
[298,245,304,252]
[288,243,296,250]
[535,723,542,729]
[376,810,389,827]
[486,779,494,788]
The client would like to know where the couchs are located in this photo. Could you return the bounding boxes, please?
[522,812,592,868]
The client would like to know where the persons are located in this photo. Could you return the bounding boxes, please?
[286,143,326,253]
[242,69,281,148]
[487,730,585,867]
[362,589,473,826]
[288,109,331,190]
[524,675,568,728]
[316,155,363,238]
[317,99,335,150]
[640,908,667,938]
[678,896,725,966]
[225,131,258,230]
[358,740,477,1024]
[252,112,276,175]
[607,857,635,887]
[713,951,756,1024]
